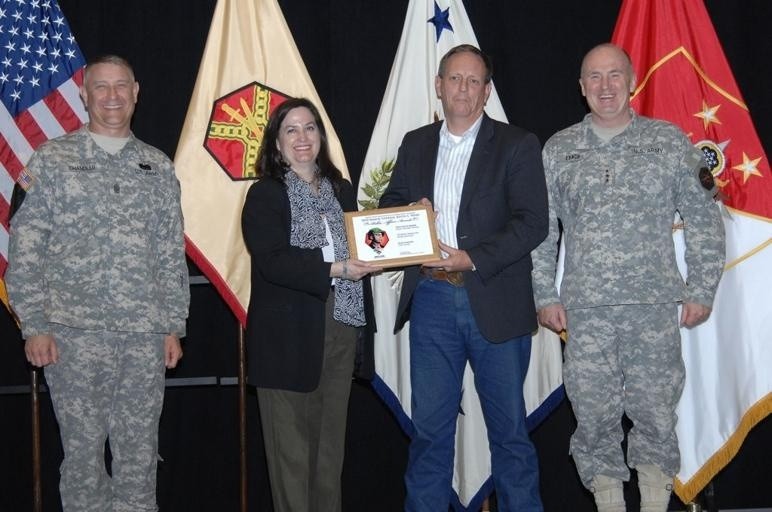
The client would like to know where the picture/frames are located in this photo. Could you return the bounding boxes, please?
[343,203,441,271]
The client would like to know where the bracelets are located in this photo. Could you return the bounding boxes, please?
[342,256,348,278]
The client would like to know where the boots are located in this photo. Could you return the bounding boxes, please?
[590,473,626,511]
[637,465,674,512]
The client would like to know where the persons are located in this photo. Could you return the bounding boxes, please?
[528,44,727,511]
[7,56,196,509]
[367,227,385,248]
[239,99,376,508]
[370,43,551,511]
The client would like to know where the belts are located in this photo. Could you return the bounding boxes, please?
[421,268,465,288]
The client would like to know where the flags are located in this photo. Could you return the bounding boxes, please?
[171,2,352,331]
[584,2,770,505]
[351,1,566,508]
[1,2,91,327]
